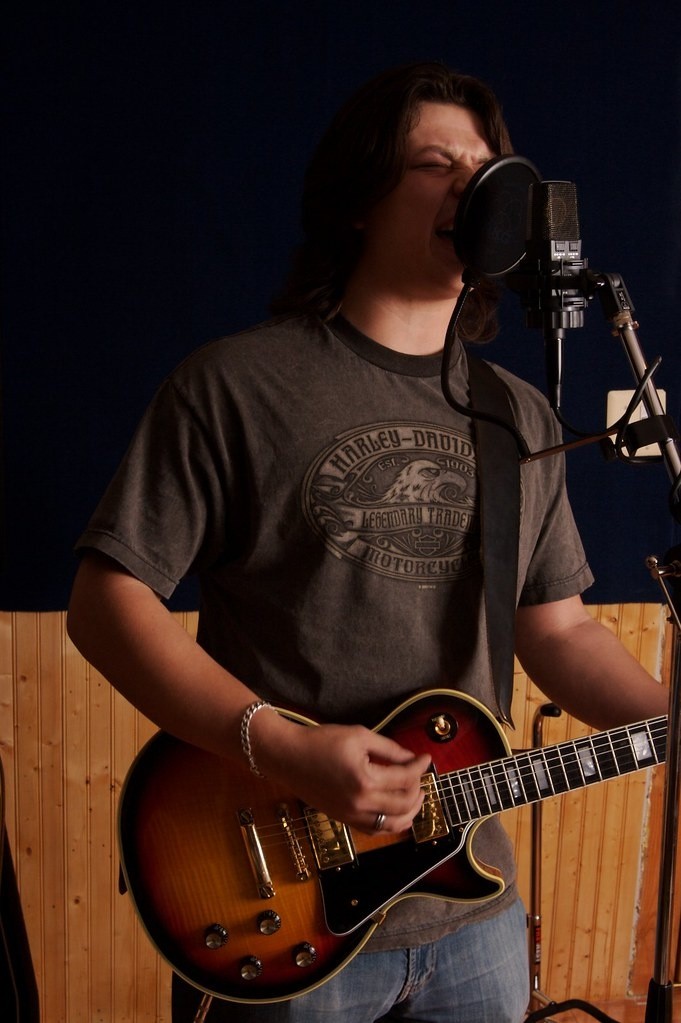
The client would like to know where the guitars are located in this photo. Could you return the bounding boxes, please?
[115,686,669,1006]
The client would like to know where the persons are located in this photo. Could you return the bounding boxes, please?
[67,70,669,1023]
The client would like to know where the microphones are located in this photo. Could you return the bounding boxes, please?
[524,180,587,408]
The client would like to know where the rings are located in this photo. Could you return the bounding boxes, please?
[375,813,385,833]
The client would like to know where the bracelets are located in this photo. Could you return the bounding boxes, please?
[241,698,272,779]
